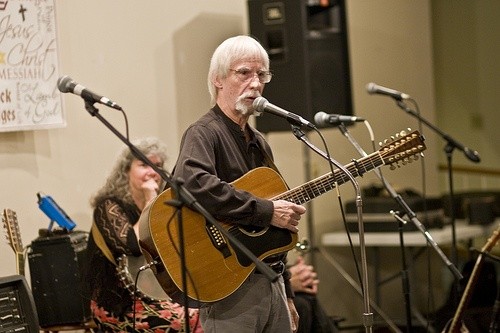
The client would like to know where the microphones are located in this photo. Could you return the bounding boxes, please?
[252,96,316,129]
[57,75,121,110]
[365,82,411,100]
[314,111,366,128]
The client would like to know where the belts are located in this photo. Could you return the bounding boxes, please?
[254,261,286,275]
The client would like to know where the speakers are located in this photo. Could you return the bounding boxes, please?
[247,0,354,133]
[28,230,92,328]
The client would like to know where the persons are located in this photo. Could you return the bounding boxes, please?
[80,144,204,333]
[287,257,340,333]
[170,34,308,333]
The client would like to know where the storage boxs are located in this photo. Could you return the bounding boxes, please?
[344,198,444,232]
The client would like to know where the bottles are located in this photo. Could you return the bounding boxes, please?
[37,191,76,232]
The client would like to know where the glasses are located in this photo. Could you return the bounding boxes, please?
[227,68,275,84]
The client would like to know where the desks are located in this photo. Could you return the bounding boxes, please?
[323,220,500,333]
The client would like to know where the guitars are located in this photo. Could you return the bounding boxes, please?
[1,206,29,276]
[136,128,426,302]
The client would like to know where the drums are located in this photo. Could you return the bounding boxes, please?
[116,253,173,302]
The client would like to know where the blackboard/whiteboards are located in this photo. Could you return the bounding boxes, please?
[0,0,67,133]
